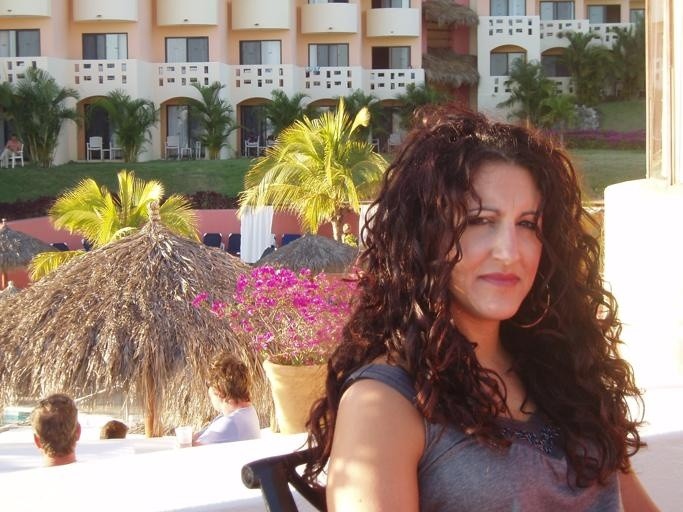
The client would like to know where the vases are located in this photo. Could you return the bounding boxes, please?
[257,353,339,438]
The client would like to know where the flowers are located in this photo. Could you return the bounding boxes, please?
[182,260,372,368]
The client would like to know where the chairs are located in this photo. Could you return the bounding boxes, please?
[239,443,332,512]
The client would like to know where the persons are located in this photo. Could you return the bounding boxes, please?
[298,100,663,511]
[0,132,21,169]
[178,353,261,447]
[99,420,130,440]
[27,391,84,469]
